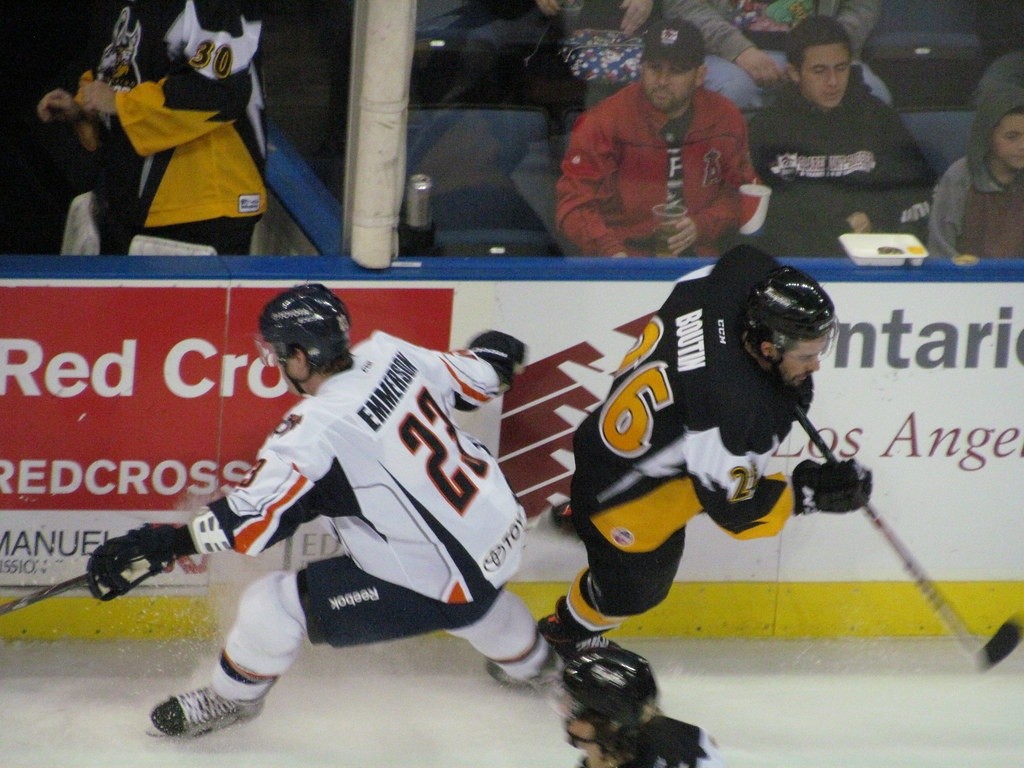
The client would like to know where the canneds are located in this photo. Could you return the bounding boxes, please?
[407,173,432,230]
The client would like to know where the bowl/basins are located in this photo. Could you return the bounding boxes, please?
[838,232,929,266]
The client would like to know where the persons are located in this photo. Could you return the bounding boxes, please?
[535,243,873,767]
[86,283,565,739]
[747,14,937,257]
[555,17,765,259]
[37,0,269,256]
[929,48,1024,258]
[412,0,893,122]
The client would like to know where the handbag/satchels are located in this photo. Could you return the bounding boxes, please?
[556,28,641,82]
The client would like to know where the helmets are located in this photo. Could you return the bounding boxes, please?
[258,284,352,366]
[559,647,657,725]
[746,265,834,361]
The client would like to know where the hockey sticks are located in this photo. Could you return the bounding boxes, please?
[792,403,1016,670]
[0,574,92,612]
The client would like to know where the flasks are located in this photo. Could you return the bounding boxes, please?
[405,175,433,229]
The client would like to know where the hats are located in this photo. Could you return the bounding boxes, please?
[640,19,706,71]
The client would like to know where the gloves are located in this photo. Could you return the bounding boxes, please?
[467,330,525,387]
[785,374,815,417]
[789,456,874,517]
[88,520,198,603]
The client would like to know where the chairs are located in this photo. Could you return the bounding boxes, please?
[408,0,991,257]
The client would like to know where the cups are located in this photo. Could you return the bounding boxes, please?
[738,184,772,238]
[559,0,585,26]
[651,204,689,258]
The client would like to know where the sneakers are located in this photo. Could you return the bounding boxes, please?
[142,678,279,740]
[535,596,620,661]
[486,644,561,693]
